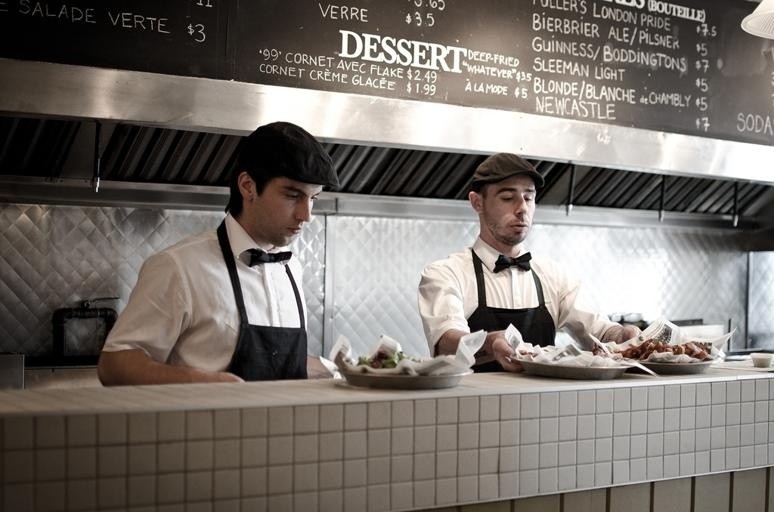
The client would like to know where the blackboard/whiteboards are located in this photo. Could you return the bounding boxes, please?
[0,0,774,147]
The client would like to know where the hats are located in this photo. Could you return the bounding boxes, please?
[472,153,545,188]
[237,121,340,189]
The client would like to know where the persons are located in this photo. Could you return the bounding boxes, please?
[416,152,644,372]
[97,122,340,384]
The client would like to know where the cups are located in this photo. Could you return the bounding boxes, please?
[749,352,772,367]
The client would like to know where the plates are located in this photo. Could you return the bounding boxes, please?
[337,369,475,387]
[508,357,633,377]
[636,360,719,375]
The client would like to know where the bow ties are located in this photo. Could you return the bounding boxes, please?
[494,252,532,272]
[249,250,292,267]
